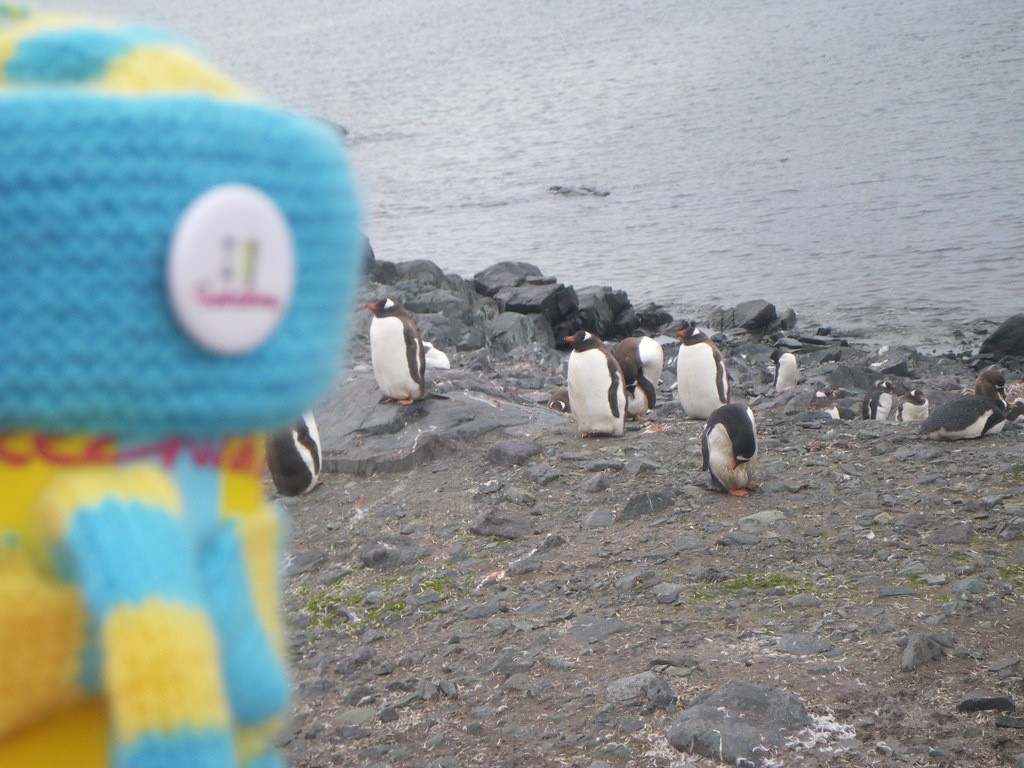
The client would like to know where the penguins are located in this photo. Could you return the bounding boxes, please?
[365,298,425,405]
[266,407,322,496]
[562,326,1024,497]
